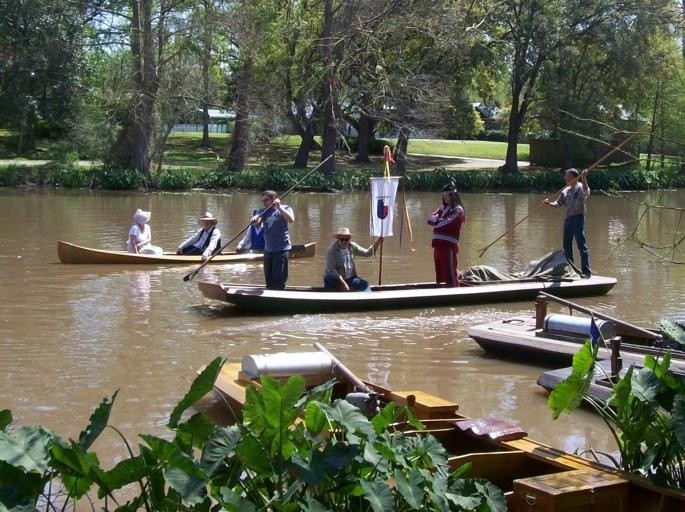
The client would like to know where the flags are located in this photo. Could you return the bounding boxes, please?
[369,177,399,237]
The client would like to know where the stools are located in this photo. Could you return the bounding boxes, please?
[386,389,459,439]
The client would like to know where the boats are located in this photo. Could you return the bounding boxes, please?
[57,239,316,265]
[537,335,684,425]
[197,341,685,511]
[198,269,618,308]
[467,291,685,387]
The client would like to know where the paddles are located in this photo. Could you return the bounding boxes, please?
[477,121,652,259]
[182,155,333,281]
[241,245,306,254]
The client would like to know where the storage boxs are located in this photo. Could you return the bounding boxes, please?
[510,466,634,511]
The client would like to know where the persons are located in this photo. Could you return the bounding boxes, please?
[426,183,465,287]
[543,167,591,278]
[236,209,264,254]
[383,145,396,177]
[324,227,384,291]
[176,212,221,262]
[249,190,295,290]
[127,208,164,256]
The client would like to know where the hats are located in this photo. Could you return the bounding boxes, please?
[331,228,353,238]
[198,212,217,225]
[132,209,151,225]
